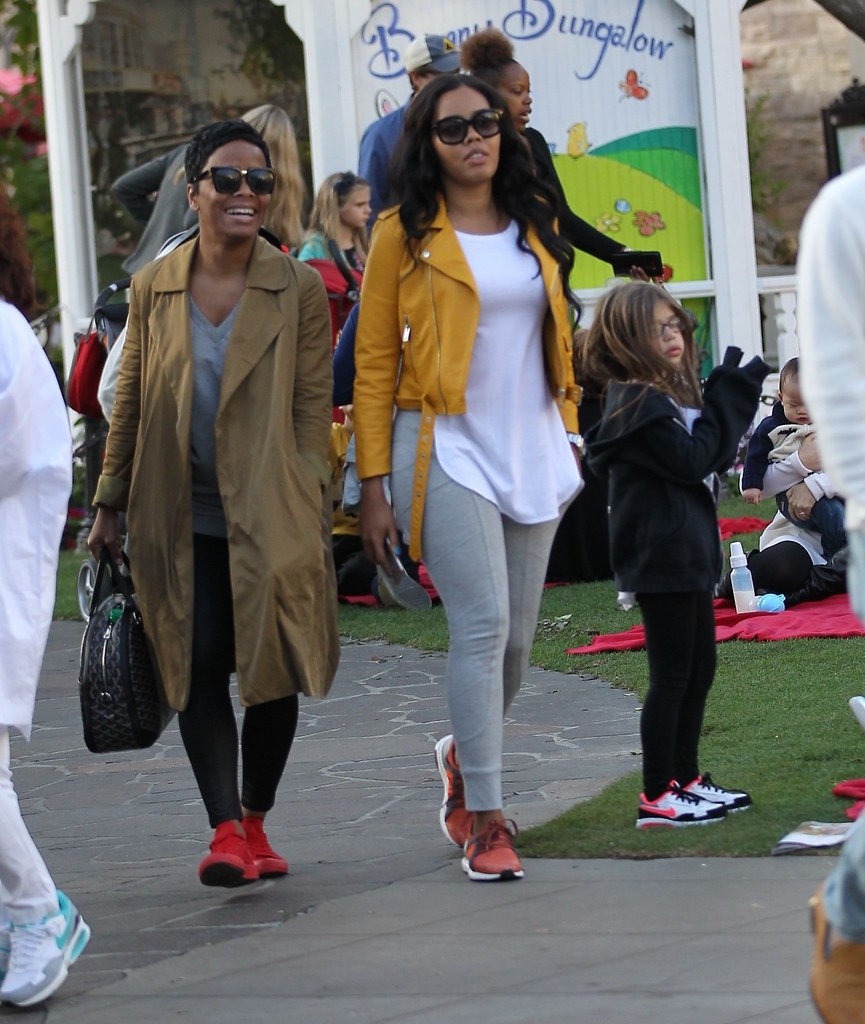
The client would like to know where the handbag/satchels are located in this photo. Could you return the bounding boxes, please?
[78,547,177,753]
[65,306,107,417]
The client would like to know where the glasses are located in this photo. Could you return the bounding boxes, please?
[189,167,277,195]
[650,318,684,337]
[430,109,503,145]
[335,171,356,196]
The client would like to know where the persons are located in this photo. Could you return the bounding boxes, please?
[462,29,651,281]
[793,160,865,1024]
[358,34,464,239]
[352,74,584,881]
[716,358,851,604]
[568,282,771,827]
[297,172,432,611]
[86,103,340,882]
[0,182,92,1009]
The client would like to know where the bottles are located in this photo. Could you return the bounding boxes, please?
[729,541,756,614]
[748,593,786,612]
[108,608,124,624]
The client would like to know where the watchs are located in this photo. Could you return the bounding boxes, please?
[567,433,587,458]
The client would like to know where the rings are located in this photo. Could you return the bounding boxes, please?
[799,512,804,515]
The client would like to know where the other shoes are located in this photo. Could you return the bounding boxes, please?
[377,557,431,611]
[807,884,865,1024]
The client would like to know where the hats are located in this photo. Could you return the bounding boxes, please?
[402,35,460,72]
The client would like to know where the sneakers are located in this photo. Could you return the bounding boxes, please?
[0,928,12,972]
[635,780,727,830]
[199,821,258,888]
[682,772,753,813]
[240,821,288,875]
[435,734,476,848]
[462,818,525,880]
[0,889,90,1006]
